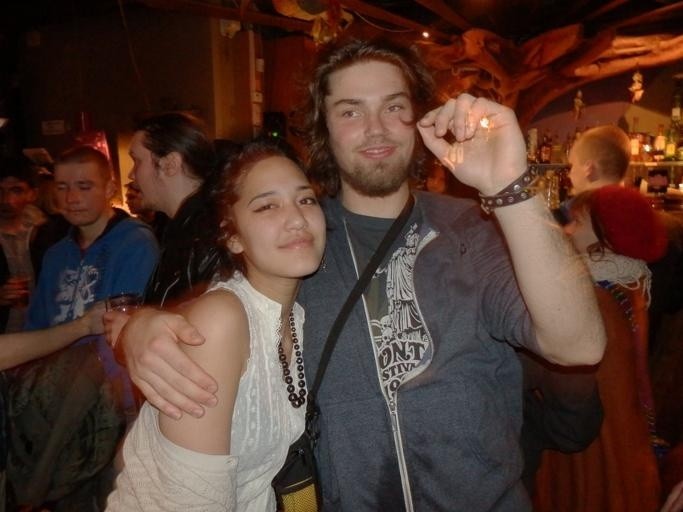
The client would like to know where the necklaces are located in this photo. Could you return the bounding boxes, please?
[276,312,308,409]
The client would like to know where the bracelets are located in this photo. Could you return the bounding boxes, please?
[476,161,545,216]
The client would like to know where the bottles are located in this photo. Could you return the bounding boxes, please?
[574,125,581,144]
[640,164,649,187]
[105,292,139,365]
[644,136,652,162]
[632,164,640,187]
[545,170,555,190]
[668,166,677,190]
[668,94,683,160]
[629,116,645,161]
[557,167,571,191]
[654,124,668,162]
[526,127,538,164]
[546,174,560,210]
[539,127,551,163]
[624,165,634,190]
[560,132,572,163]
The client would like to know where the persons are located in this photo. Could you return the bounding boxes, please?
[510,350,603,498]
[421,159,457,193]
[211,139,245,162]
[533,185,666,512]
[0,154,69,331]
[303,140,338,196]
[262,111,300,162]
[106,136,340,512]
[124,182,158,224]
[1,299,115,371]
[660,481,683,510]
[550,125,683,348]
[112,36,607,509]
[21,146,159,417]
[23,149,55,183]
[103,111,218,350]
[535,142,553,162]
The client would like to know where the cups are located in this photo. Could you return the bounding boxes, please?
[9,276,31,310]
[108,294,140,317]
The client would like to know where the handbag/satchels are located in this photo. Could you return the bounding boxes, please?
[268,192,415,510]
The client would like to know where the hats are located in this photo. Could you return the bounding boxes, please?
[592,184,669,262]
